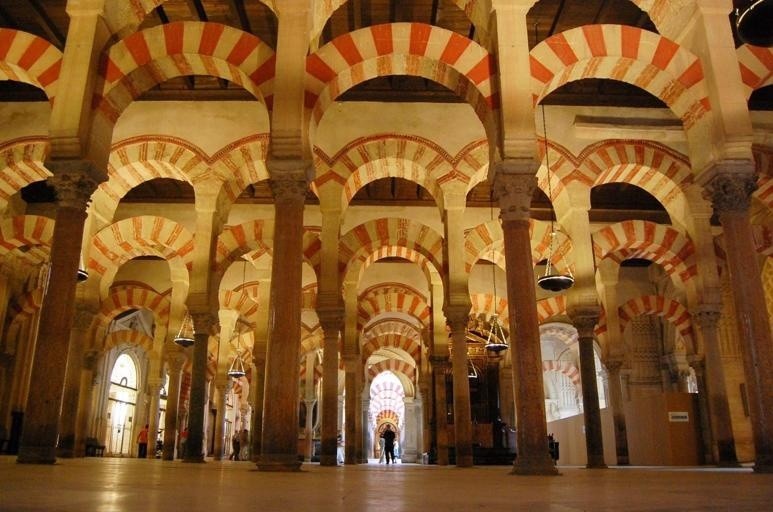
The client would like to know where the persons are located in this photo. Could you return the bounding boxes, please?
[228,428,250,460]
[177,427,189,458]
[136,424,149,457]
[379,424,397,464]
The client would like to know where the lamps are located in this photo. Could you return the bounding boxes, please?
[483,189,509,351]
[467,354,478,380]
[226,260,247,379]
[76,250,88,282]
[172,312,196,348]
[532,21,573,292]
